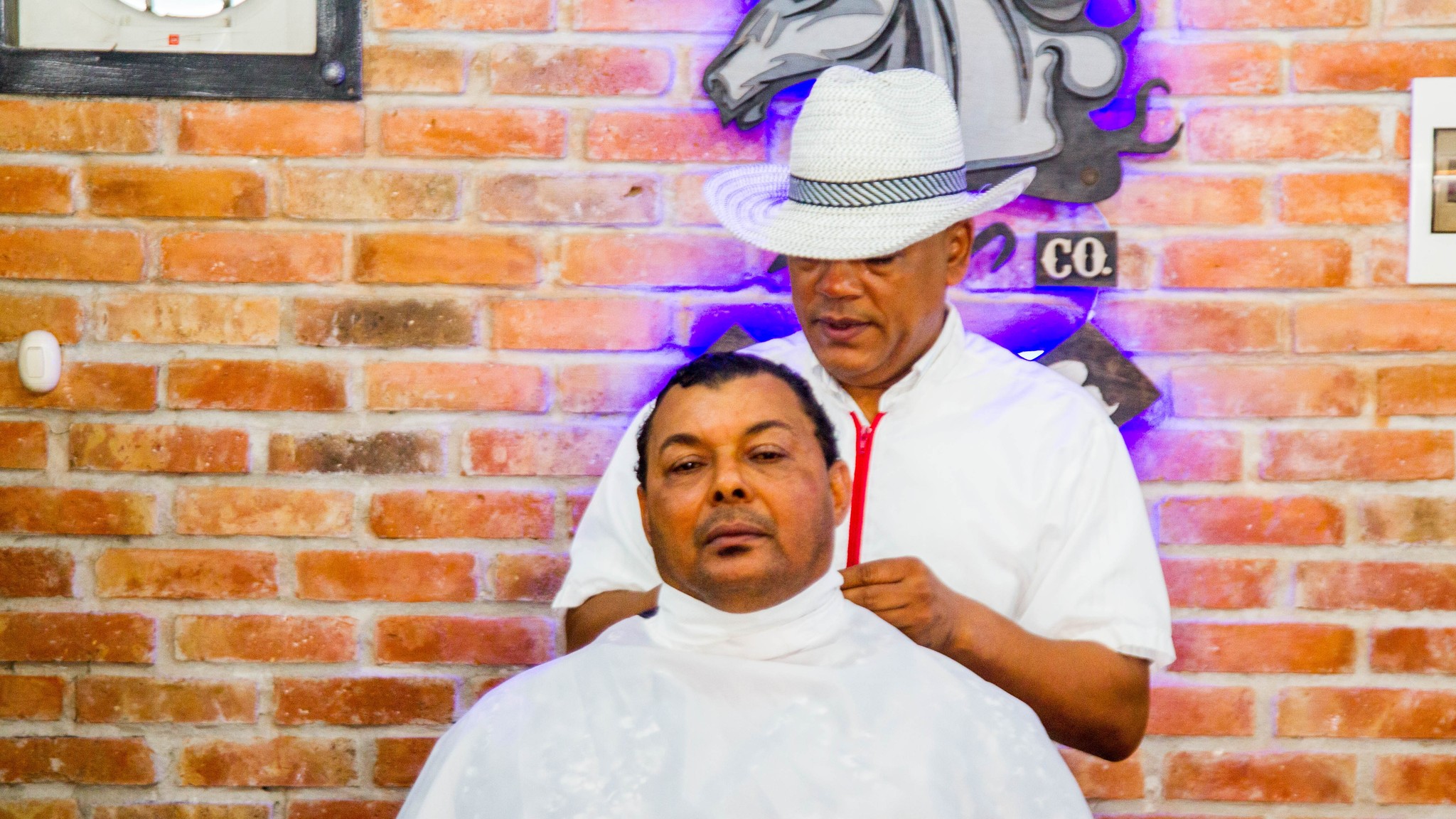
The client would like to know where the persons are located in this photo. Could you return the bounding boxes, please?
[397,352,1092,819]
[550,66,1177,764]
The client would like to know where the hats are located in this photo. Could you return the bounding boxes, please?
[702,62,1035,260]
